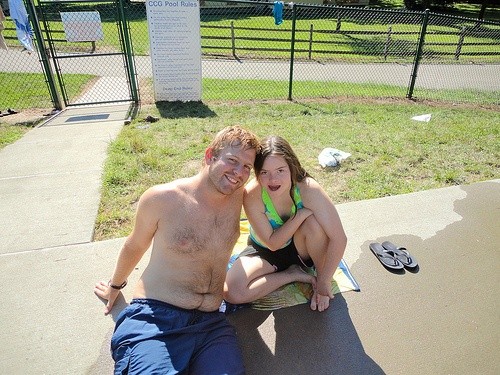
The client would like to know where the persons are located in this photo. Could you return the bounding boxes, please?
[223,136,347,311]
[93,126,260,375]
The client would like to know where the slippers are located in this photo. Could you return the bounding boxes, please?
[382,241,418,267]
[369,242,404,269]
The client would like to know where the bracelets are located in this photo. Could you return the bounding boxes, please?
[108,280,127,290]
